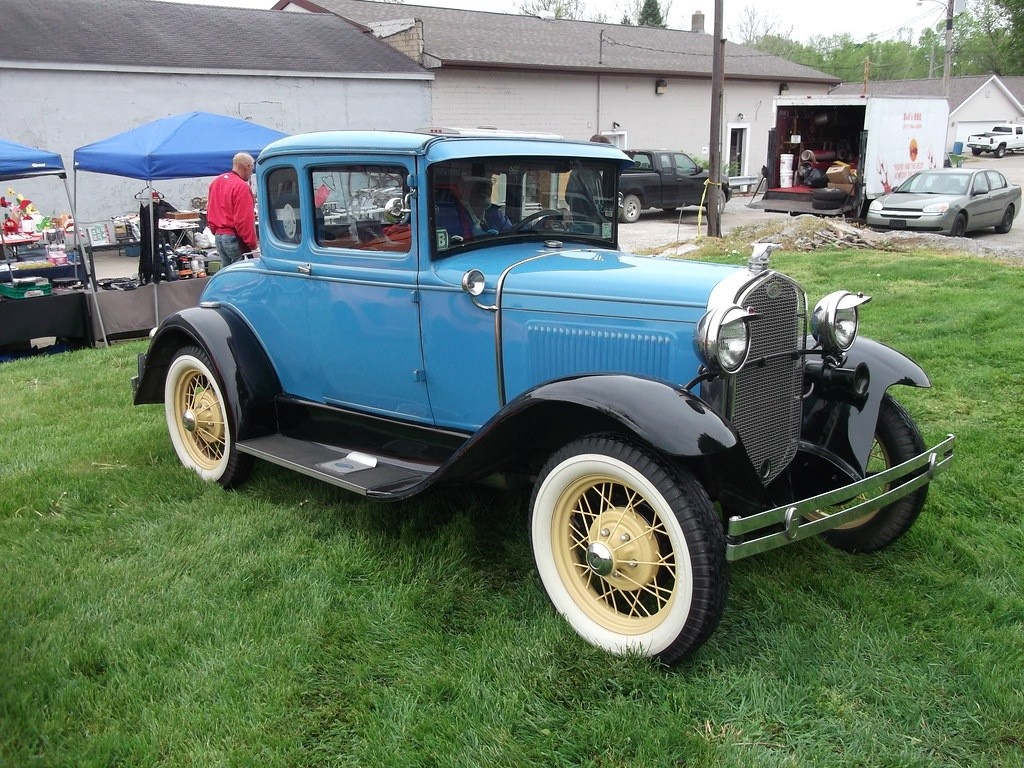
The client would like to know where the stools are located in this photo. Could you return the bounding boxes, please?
[116,237,134,256]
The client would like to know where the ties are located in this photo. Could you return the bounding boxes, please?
[479,220,489,232]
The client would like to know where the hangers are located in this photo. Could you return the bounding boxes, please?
[133,179,163,199]
[320,171,336,191]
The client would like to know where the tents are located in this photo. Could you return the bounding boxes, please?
[0,139,109,349]
[73,111,293,328]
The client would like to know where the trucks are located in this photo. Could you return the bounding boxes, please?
[746,95,963,219]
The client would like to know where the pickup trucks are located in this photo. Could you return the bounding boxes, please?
[616,148,733,223]
[967,123,1024,159]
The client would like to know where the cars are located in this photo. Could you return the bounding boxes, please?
[866,168,1022,237]
[127,128,957,671]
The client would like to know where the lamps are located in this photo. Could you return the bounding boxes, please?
[779,84,789,95]
[656,80,667,94]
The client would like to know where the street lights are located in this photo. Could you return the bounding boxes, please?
[917,0,953,98]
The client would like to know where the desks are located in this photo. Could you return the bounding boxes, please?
[0,235,38,262]
[0,259,90,284]
[0,274,214,362]
[157,218,201,250]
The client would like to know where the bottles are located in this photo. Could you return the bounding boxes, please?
[48,241,67,265]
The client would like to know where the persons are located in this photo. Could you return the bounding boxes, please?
[565,135,621,251]
[433,158,533,242]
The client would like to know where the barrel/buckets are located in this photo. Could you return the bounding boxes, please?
[953,141,963,155]
[780,170,794,188]
[780,154,794,170]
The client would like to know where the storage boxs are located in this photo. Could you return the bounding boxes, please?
[825,161,854,197]
